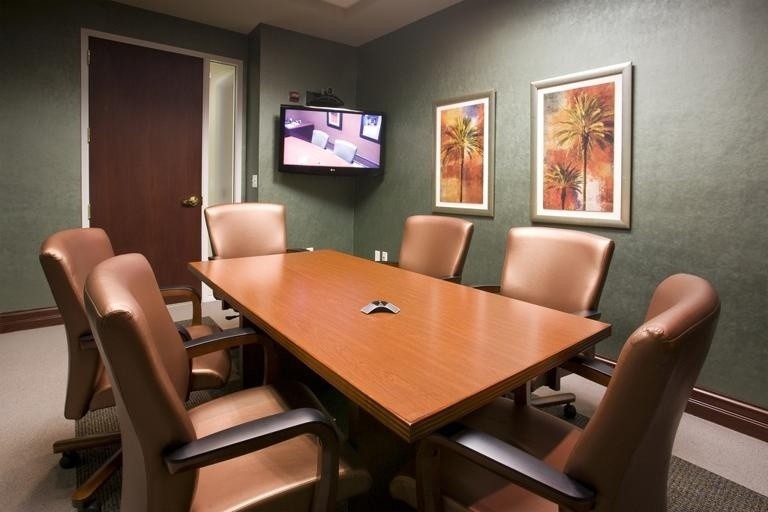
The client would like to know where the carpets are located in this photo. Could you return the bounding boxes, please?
[74,324,768,511]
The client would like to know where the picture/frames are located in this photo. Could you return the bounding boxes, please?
[431,88,495,218]
[326,112,342,130]
[529,60,631,230]
[358,114,382,144]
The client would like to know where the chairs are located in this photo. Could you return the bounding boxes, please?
[333,139,357,164]
[82,253,372,512]
[373,214,474,284]
[203,202,310,310]
[311,129,329,149]
[40,226,232,512]
[401,271,719,512]
[465,225,614,444]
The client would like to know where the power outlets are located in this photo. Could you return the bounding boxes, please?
[374,250,380,261]
[382,251,388,262]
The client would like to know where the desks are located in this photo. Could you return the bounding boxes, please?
[283,136,355,167]
[186,247,611,512]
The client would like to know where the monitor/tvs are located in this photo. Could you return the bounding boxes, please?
[278,103,386,177]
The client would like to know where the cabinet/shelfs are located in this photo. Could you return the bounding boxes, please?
[284,121,314,143]
